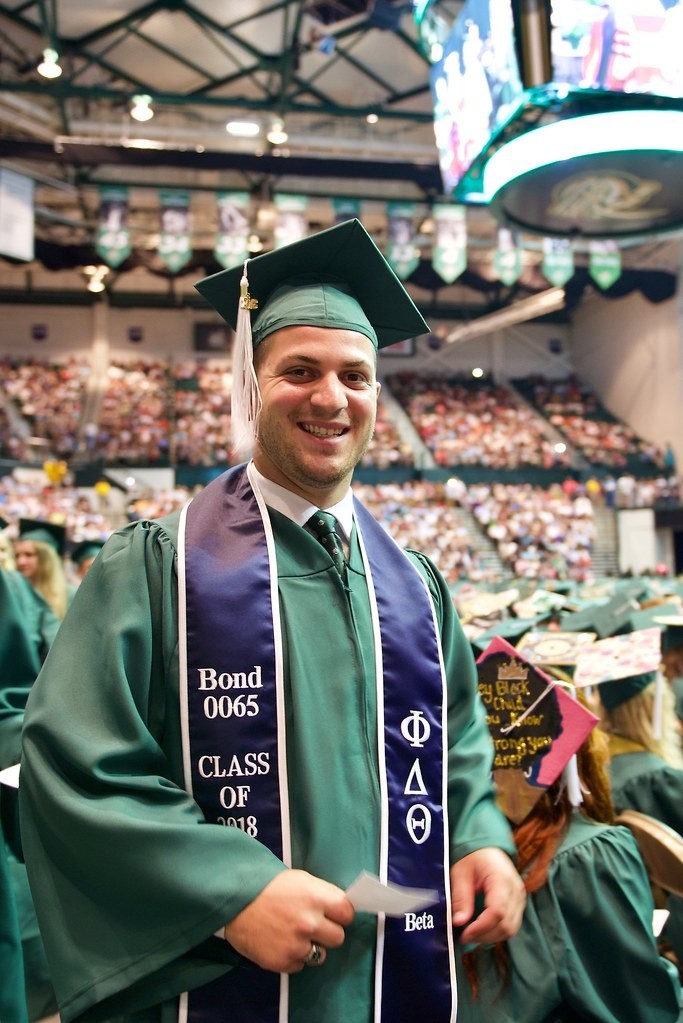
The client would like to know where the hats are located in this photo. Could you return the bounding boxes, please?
[0,512,683,985]
[192,217,433,462]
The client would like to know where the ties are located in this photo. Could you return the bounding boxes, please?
[313,512,346,580]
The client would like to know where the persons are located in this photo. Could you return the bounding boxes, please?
[0,355,250,1023]
[351,370,683,1023]
[19,218,526,1023]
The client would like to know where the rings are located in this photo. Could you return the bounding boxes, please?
[303,943,321,964]
